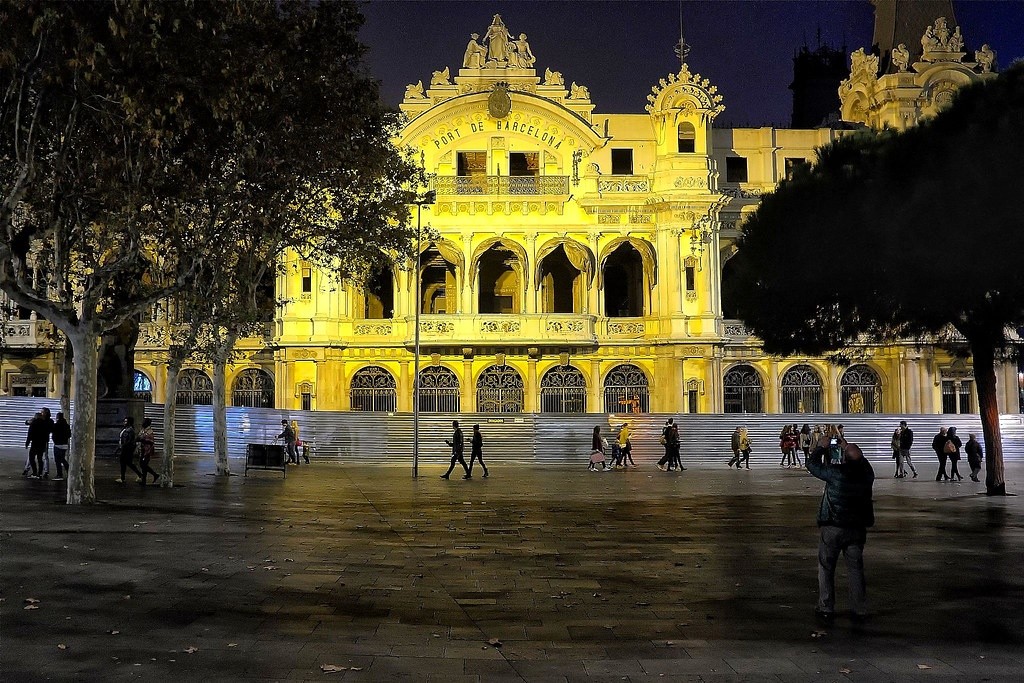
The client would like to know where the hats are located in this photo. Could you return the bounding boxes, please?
[948,427,957,435]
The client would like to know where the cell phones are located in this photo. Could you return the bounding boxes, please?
[830,438,839,445]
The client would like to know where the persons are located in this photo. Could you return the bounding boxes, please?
[891,421,919,478]
[587,423,640,472]
[439,420,473,479]
[21,407,72,481]
[654,418,687,472]
[462,424,489,479]
[779,424,845,470]
[727,426,752,471]
[115,417,160,483]
[806,425,875,643]
[932,427,963,482]
[965,434,983,482]
[275,419,311,466]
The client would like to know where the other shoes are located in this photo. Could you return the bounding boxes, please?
[288,462,297,465]
[815,608,836,622]
[656,464,687,472]
[440,473,449,480]
[115,479,123,483]
[139,479,146,485]
[135,477,142,483]
[461,474,473,480]
[152,473,160,483]
[43,472,49,477]
[28,473,39,479]
[482,471,488,477]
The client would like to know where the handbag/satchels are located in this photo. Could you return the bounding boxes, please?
[591,451,605,463]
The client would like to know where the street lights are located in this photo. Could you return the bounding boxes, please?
[394,189,437,477]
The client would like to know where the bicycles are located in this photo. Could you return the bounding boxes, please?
[269,436,290,464]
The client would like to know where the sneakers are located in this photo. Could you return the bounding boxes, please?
[602,467,610,472]
[52,475,63,480]
[591,468,598,472]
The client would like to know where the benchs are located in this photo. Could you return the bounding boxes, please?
[245,444,287,479]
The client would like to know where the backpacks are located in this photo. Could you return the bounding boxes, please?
[944,436,959,453]
